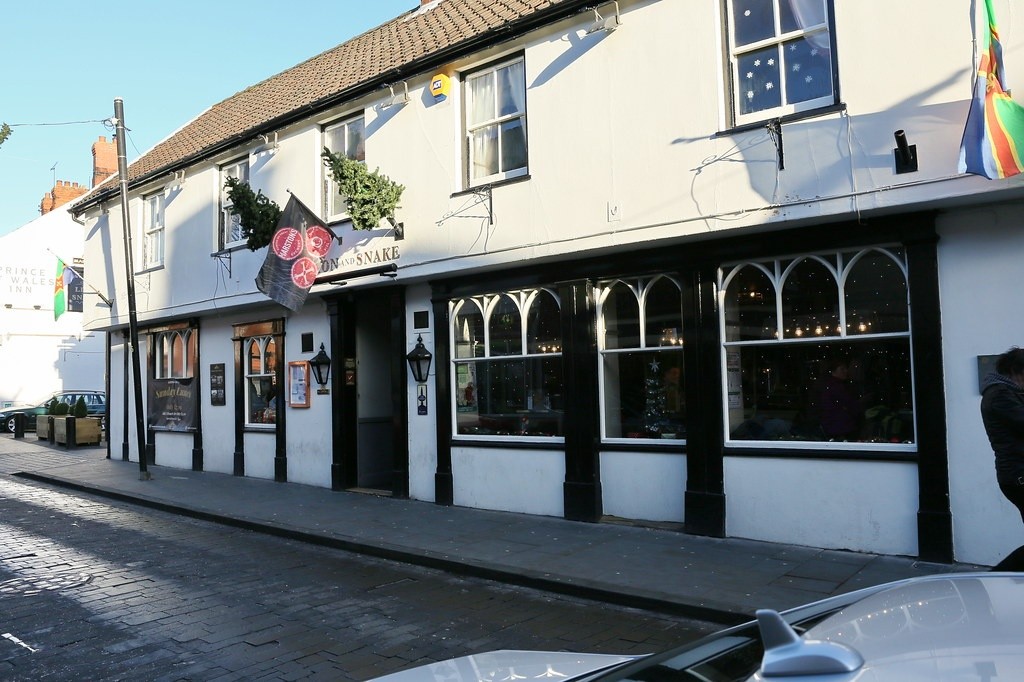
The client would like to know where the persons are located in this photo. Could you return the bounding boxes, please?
[979,345,1024,572]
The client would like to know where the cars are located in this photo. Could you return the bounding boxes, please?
[0,390,106,434]
[367,571,1024,681]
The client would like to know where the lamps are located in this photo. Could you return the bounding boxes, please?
[92,201,109,216]
[407,333,433,381]
[163,169,186,190]
[253,131,280,156]
[308,342,332,384]
[379,80,411,109]
[579,0,623,35]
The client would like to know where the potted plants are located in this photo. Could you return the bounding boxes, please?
[36,395,101,443]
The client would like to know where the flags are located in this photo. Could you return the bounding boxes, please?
[54,258,67,321]
[254,193,336,316]
[956,1,1024,179]
